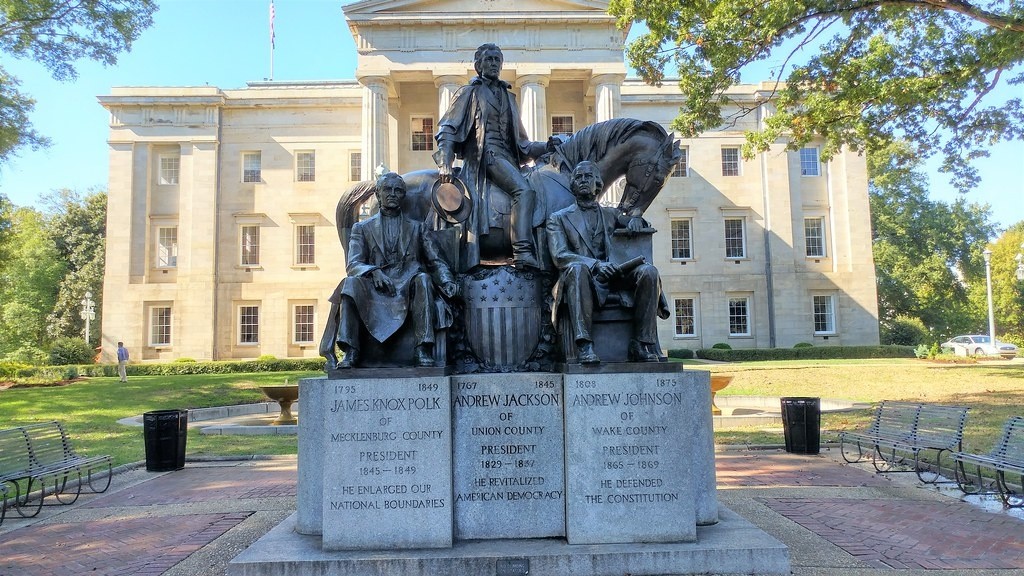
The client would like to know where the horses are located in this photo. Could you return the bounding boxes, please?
[320,118,683,370]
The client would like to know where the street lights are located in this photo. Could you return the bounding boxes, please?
[80,291,96,343]
[983,250,996,355]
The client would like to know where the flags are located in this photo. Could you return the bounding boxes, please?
[269,2,276,50]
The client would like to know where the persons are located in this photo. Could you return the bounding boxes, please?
[332,172,462,369]
[547,161,663,365]
[433,43,563,271]
[117,342,130,383]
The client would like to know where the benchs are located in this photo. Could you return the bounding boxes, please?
[0,420,115,529]
[836,399,973,486]
[948,412,1024,508]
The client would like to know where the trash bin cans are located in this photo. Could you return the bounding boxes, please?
[143,409,188,472]
[781,396,820,455]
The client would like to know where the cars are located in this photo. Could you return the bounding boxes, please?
[940,335,1018,360]
